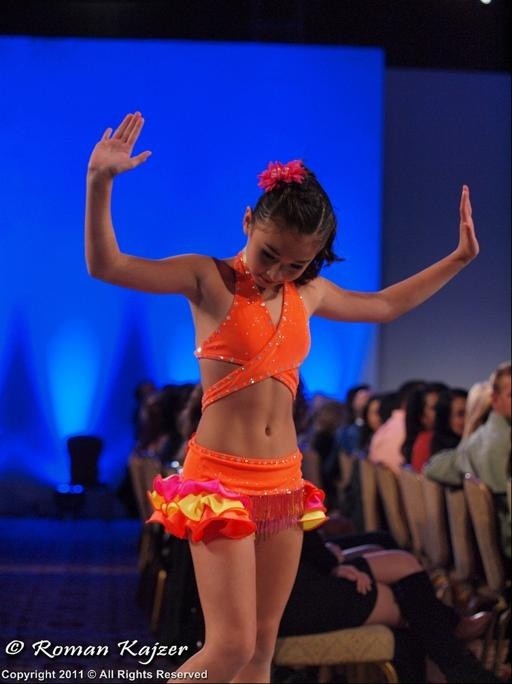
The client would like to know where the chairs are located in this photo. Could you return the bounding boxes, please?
[67,435,512,684]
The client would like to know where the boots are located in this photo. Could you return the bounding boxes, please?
[391,571,508,684]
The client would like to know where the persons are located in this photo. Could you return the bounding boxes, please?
[134,363,512,684]
[85,109,481,684]
